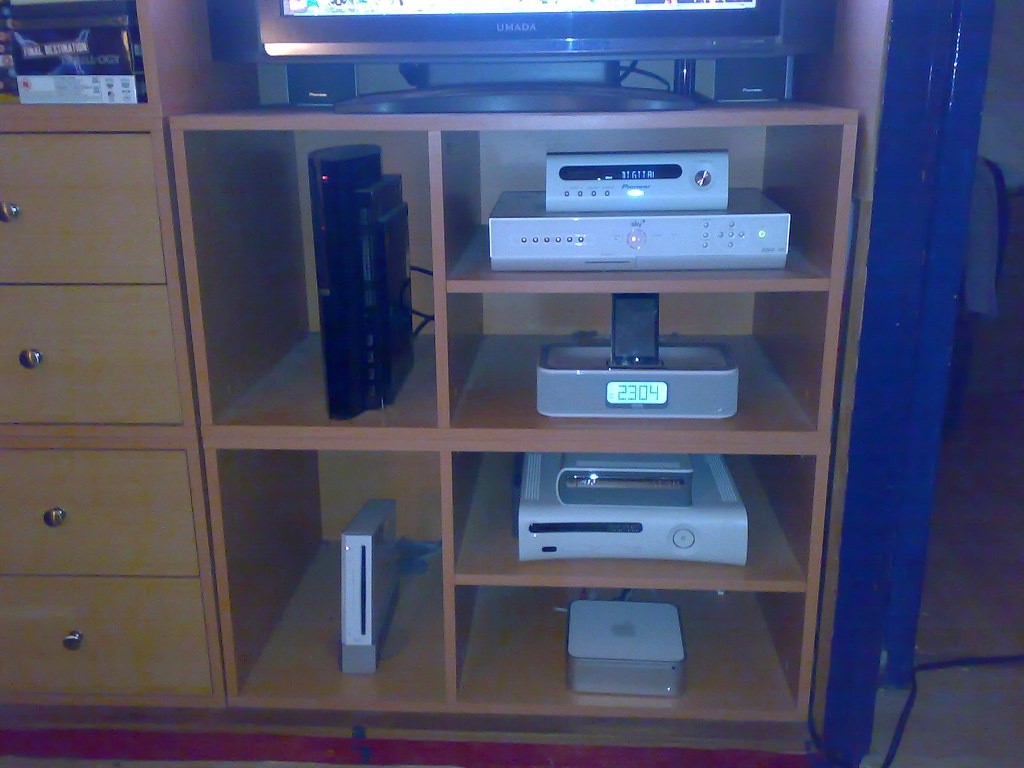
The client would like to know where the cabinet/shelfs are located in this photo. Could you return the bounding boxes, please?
[0,0,893,768]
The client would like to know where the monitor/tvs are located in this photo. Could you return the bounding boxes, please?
[256,0,838,118]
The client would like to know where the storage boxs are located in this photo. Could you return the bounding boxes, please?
[16,74,138,104]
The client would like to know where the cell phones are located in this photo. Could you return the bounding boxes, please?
[610,294,659,369]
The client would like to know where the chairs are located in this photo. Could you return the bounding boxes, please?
[944,161,1009,432]
